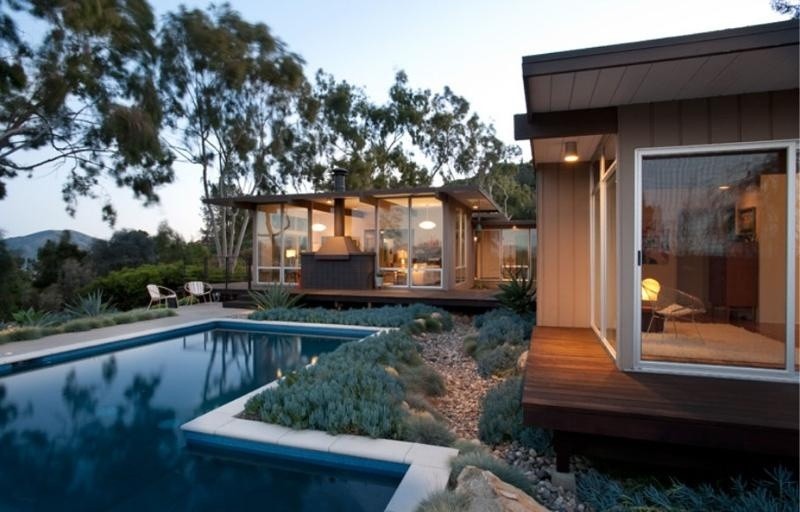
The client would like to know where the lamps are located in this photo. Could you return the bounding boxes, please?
[563,142,579,162]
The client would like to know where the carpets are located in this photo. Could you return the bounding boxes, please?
[640,316,799,367]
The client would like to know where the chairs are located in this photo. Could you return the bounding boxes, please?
[641,282,706,337]
[144,278,214,312]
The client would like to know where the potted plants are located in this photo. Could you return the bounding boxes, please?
[375,272,385,288]
